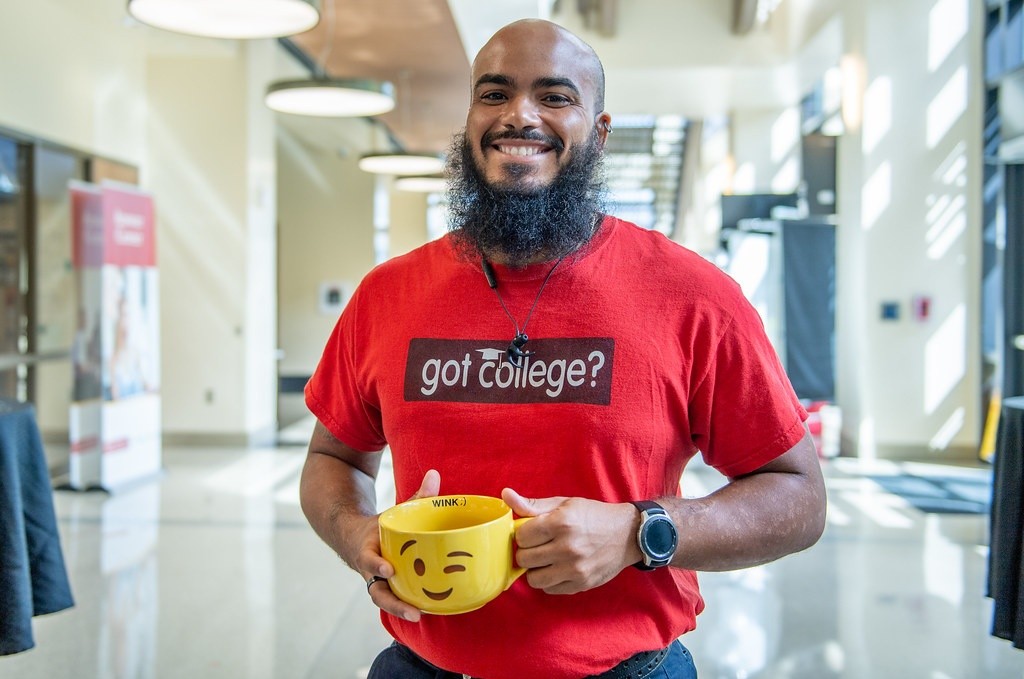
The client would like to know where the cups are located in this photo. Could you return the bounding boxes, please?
[377,494,537,616]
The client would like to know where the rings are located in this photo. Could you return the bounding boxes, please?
[367,576,389,594]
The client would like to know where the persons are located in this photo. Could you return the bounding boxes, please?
[298,19,825,679]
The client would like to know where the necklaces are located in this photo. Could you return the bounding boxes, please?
[476,243,577,369]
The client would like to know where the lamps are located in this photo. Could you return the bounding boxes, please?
[127,0,455,192]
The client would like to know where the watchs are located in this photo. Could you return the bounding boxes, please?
[629,499,679,571]
